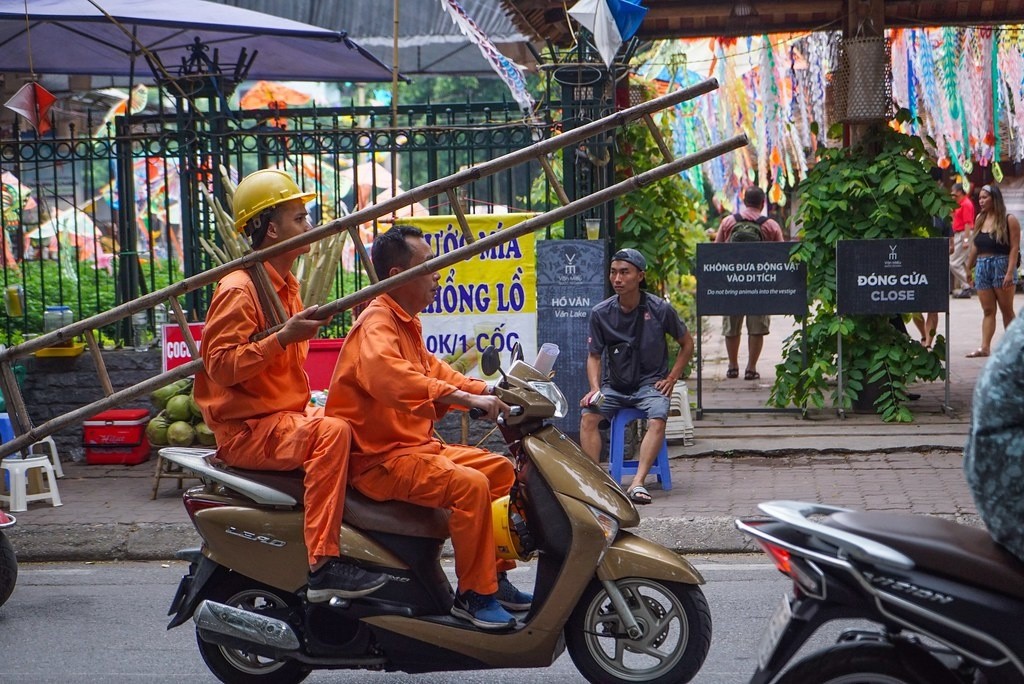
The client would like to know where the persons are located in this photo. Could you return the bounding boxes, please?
[962,306,1024,560]
[325,226,534,629]
[194,169,389,602]
[580,248,694,505]
[714,186,784,380]
[949,180,1020,358]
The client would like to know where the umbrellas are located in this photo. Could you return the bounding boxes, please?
[0,0,413,116]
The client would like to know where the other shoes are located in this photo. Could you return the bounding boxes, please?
[965,348,990,357]
[898,393,921,401]
[727,368,738,378]
[745,370,760,380]
[955,290,970,298]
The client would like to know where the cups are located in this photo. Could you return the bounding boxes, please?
[474,321,505,380]
[585,218,601,240]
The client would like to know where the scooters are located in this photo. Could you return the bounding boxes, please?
[733,498,1024,684]
[152,340,714,684]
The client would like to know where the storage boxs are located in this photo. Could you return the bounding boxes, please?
[80,408,151,465]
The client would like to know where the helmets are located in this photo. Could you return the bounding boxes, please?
[233,169,318,231]
[491,495,533,562]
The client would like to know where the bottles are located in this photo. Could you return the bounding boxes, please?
[44,306,73,348]
[309,389,328,407]
[511,511,531,549]
[131,308,148,353]
[154,303,167,347]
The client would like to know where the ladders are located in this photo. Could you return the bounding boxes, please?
[0,79,749,455]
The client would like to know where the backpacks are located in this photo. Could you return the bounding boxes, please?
[729,214,774,242]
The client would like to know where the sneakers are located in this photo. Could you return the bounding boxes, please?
[450,588,517,629]
[307,558,388,603]
[492,579,533,611]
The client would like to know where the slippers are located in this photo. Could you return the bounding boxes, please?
[627,486,652,503]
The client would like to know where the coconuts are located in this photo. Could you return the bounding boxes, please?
[146,378,217,447]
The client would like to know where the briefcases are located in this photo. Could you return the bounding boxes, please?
[81,410,151,465]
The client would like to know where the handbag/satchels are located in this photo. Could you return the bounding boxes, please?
[608,342,640,396]
[1017,252,1021,269]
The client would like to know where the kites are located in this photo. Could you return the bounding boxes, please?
[1,80,429,285]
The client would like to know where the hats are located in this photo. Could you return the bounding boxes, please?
[611,248,646,289]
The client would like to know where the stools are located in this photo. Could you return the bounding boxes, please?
[609,379,694,491]
[0,412,65,512]
[150,443,217,500]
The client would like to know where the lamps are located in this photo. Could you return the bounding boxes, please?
[729,0,758,18]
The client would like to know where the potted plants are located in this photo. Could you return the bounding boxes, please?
[766,106,962,422]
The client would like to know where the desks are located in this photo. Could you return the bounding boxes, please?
[433,405,499,448]
